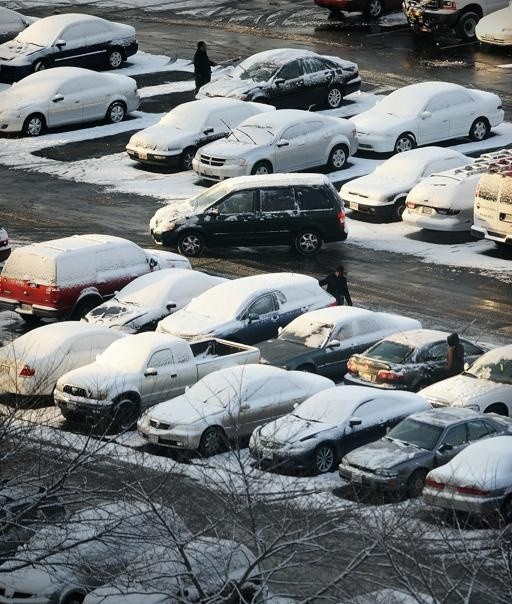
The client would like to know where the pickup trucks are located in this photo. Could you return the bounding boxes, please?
[58,329,258,422]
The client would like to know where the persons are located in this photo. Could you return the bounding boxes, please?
[319,265,353,307]
[192,40,219,100]
[444,331,465,378]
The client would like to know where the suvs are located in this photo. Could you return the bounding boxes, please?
[147,171,349,249]
[416,2,493,40]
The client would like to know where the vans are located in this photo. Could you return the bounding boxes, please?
[476,148,512,252]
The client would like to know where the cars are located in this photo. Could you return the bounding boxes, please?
[313,0,396,20]
[478,4,512,47]
[428,437,511,511]
[251,379,433,470]
[130,36,357,169]
[0,486,267,598]
[343,409,511,500]
[346,78,502,231]
[138,359,324,444]
[0,8,128,130]
[3,315,128,393]
